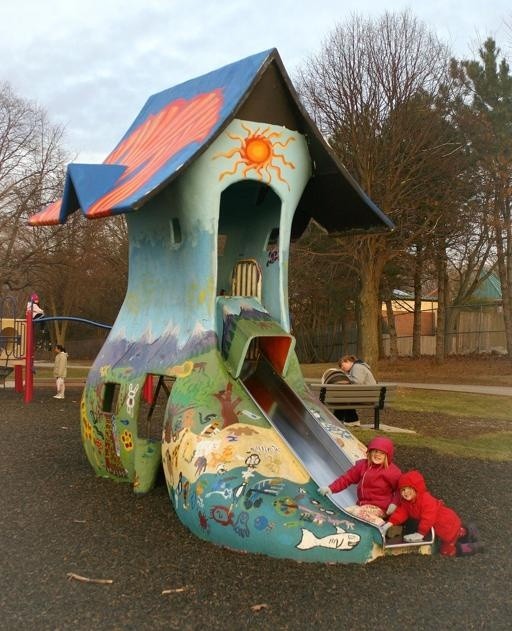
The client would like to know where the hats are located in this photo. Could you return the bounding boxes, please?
[30,293,39,301]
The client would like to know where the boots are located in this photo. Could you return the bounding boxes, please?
[454,522,486,558]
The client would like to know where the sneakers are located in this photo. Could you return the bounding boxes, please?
[386,524,404,539]
[53,393,64,399]
[342,419,361,427]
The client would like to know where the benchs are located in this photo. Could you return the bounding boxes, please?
[310,384,398,430]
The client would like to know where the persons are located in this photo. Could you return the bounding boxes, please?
[379,470,486,557]
[26,293,49,334]
[316,433,405,528]
[321,355,379,426]
[52,344,69,398]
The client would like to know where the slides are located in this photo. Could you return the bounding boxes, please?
[236,336,435,549]
